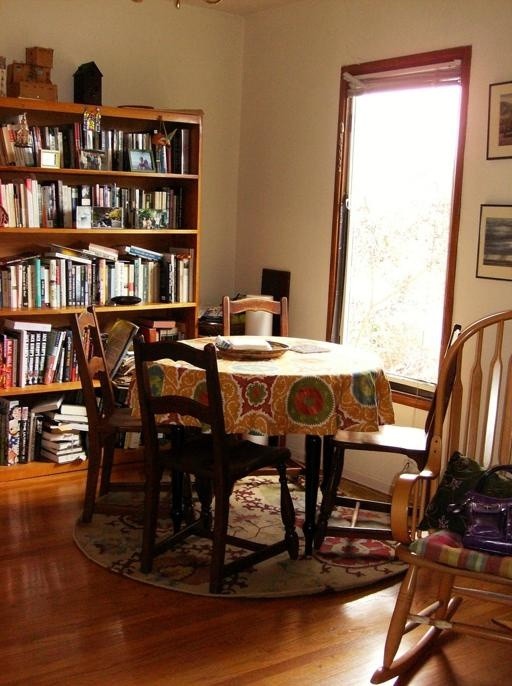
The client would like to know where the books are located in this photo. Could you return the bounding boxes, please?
[0,393,130,466]
[0,240,195,309]
[0,178,183,230]
[0,318,187,389]
[0,113,190,176]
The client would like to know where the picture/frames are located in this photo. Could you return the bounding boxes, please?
[475,204,512,282]
[486,81,512,160]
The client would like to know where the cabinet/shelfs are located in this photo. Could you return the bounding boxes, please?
[0,96,205,483]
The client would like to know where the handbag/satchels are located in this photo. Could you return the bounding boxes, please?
[461,491,512,556]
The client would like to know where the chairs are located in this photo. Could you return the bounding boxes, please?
[313,324,461,550]
[132,341,298,594]
[370,309,512,684]
[71,307,202,523]
[221,297,308,487]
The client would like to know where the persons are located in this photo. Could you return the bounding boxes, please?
[137,157,147,169]
[142,215,159,229]
[98,212,112,227]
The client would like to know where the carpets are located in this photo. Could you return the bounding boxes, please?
[72,468,412,598]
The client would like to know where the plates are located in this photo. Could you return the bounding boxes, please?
[215,341,290,360]
